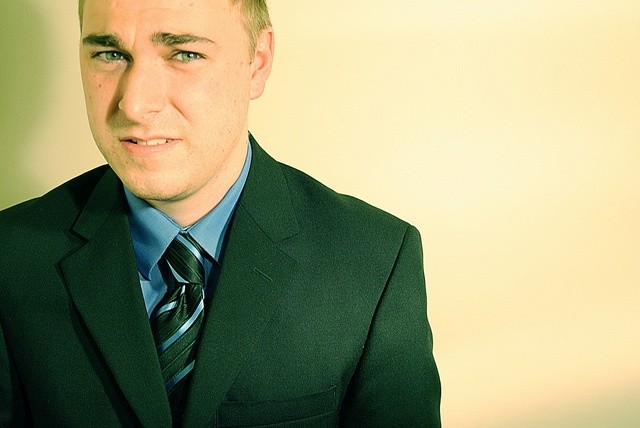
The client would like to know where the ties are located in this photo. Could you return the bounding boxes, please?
[149,232,207,426]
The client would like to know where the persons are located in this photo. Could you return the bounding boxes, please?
[1,0,441,426]
